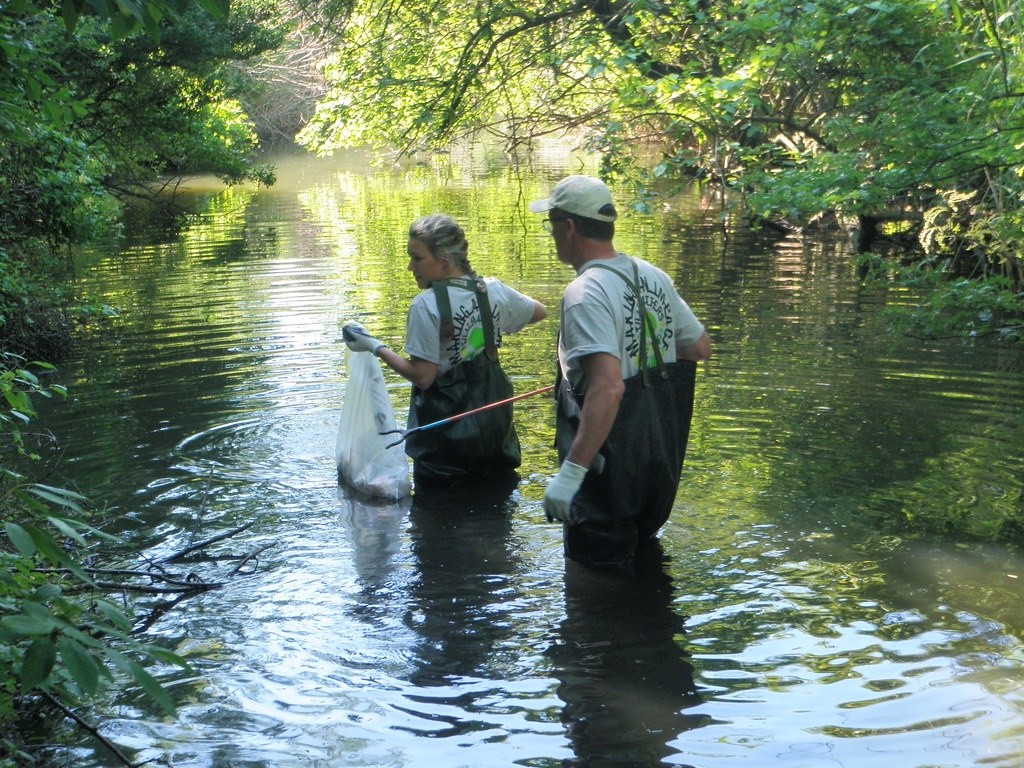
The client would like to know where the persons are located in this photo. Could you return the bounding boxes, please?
[340,214,549,513]
[527,175,712,564]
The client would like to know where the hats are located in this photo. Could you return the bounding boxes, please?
[528,175,617,223]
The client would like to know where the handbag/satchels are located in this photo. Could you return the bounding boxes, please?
[336,319,411,500]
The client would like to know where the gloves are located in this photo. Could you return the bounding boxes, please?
[542,460,589,524]
[342,320,388,357]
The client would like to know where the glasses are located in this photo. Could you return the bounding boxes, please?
[542,217,568,231]
